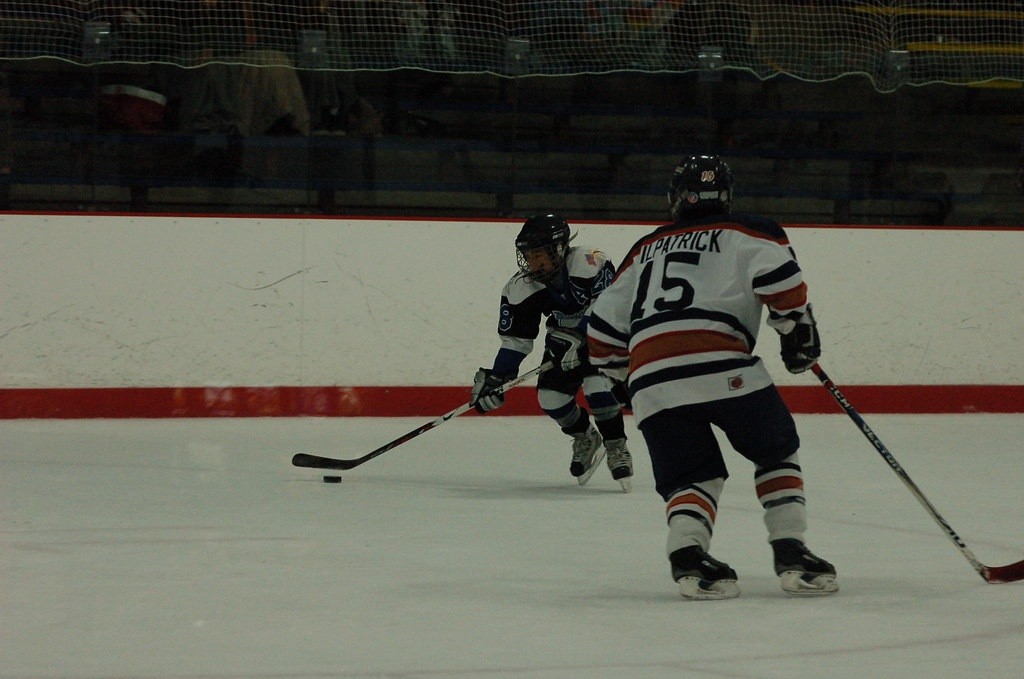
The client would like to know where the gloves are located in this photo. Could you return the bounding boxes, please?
[466,365,507,416]
[772,304,823,373]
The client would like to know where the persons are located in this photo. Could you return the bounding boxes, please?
[587,153,839,601]
[471,213,633,493]
[339,71,387,138]
[96,8,185,135]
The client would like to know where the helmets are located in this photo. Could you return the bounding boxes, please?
[514,208,572,249]
[668,154,735,205]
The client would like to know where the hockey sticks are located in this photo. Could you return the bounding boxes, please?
[290,357,554,471]
[810,362,1024,586]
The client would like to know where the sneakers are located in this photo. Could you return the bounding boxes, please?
[567,422,608,485]
[602,436,634,494]
[769,539,839,594]
[668,545,741,599]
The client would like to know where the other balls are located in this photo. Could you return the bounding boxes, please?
[323,474,342,483]
